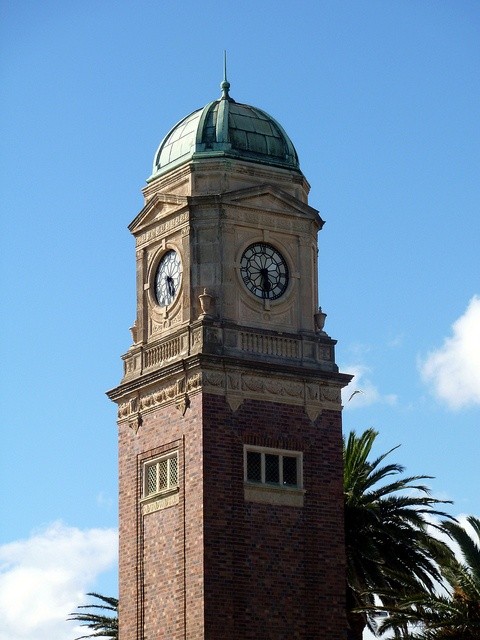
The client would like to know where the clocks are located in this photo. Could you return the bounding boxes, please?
[236,237,296,305]
[146,243,185,315]
